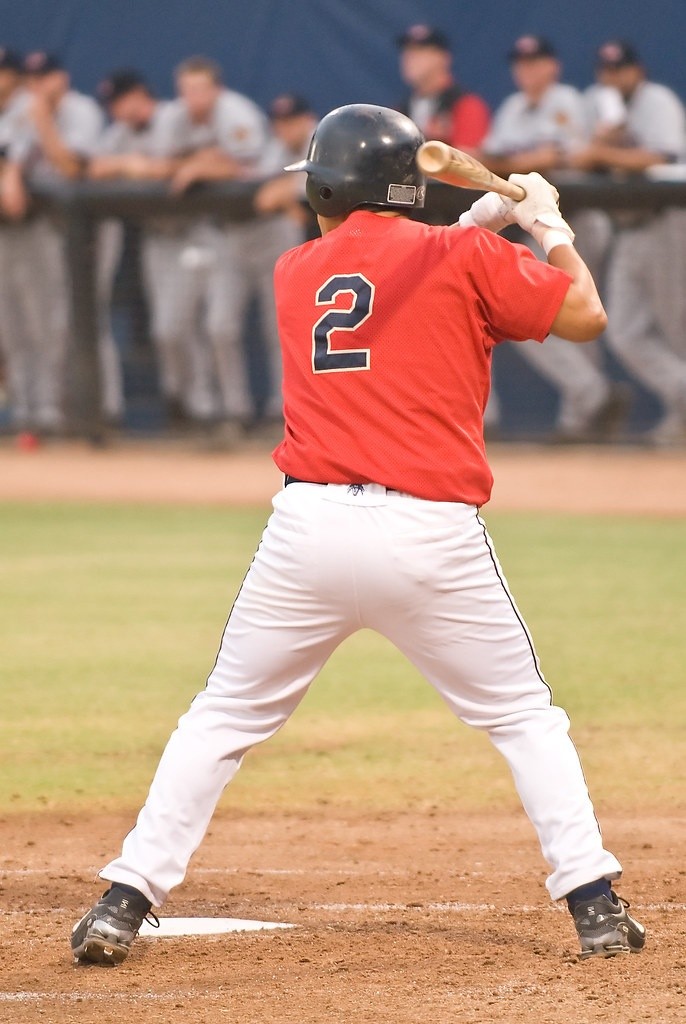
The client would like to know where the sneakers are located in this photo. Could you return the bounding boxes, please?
[70,887,160,965]
[572,890,646,957]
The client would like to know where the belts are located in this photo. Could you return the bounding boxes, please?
[285,474,397,495]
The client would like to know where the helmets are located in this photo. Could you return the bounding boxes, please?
[283,104,428,218]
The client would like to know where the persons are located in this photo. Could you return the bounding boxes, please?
[1,46,322,453]
[382,21,686,448]
[72,103,647,967]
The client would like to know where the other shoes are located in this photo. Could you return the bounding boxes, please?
[599,382,635,436]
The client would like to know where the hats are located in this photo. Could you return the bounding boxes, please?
[595,42,634,69]
[1,47,22,72]
[20,49,59,78]
[95,68,143,103]
[398,25,452,49]
[510,37,554,58]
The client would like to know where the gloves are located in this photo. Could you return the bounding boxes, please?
[498,171,574,249]
[459,191,516,236]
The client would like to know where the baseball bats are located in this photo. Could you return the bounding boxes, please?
[412,135,564,213]
